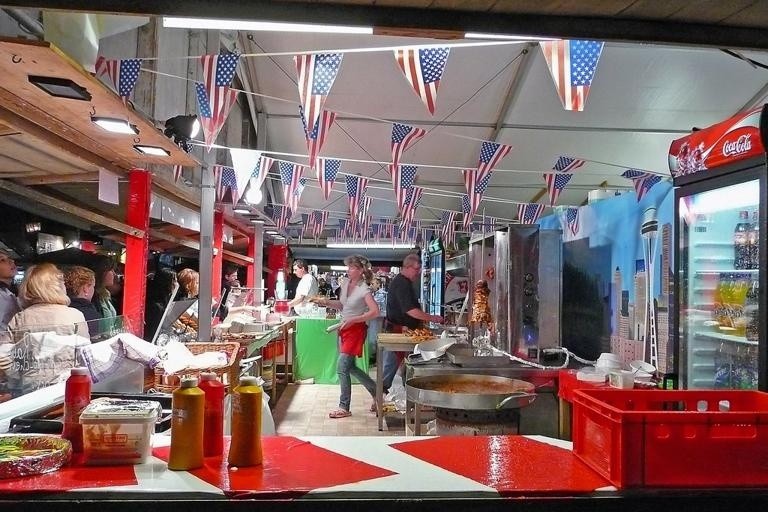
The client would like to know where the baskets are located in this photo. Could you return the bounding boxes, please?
[155,342,240,393]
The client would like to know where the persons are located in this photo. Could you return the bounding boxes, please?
[381,253,445,396]
[286,256,319,315]
[0,245,243,404]
[317,271,393,368]
[299,254,377,419]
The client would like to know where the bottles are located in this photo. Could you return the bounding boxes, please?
[747,212,760,269]
[713,273,759,391]
[733,211,750,270]
[59,368,91,452]
[166,378,205,471]
[227,377,263,468]
[197,373,224,457]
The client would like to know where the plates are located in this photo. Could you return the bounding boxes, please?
[0,432,72,478]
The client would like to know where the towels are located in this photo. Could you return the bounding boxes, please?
[75,332,168,384]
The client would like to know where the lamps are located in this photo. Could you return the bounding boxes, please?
[163,113,199,153]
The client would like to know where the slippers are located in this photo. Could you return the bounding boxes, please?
[329,410,351,417]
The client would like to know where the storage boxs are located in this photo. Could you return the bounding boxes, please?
[79,400,162,467]
[572,390,768,489]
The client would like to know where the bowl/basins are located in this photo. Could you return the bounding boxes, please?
[629,360,656,376]
[594,352,620,371]
